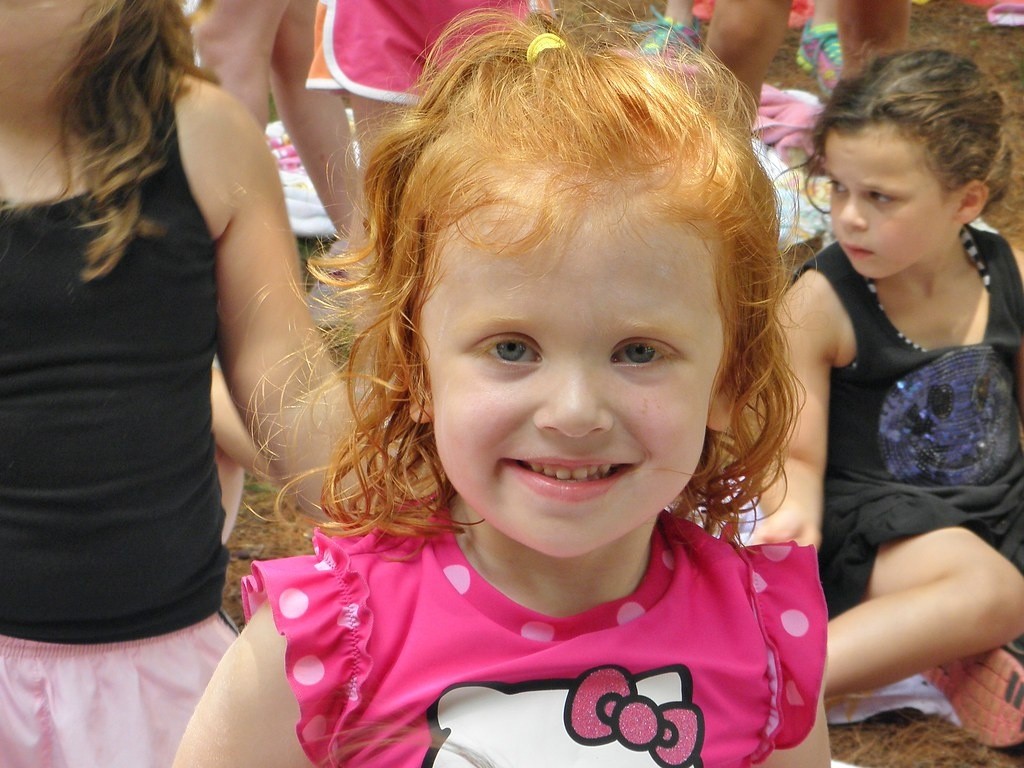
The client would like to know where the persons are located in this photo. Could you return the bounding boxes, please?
[172,4,833,768]
[0,0,405,768]
[752,43,1024,746]
[193,2,923,543]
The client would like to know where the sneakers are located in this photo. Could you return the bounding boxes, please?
[629,5,702,56]
[795,19,844,99]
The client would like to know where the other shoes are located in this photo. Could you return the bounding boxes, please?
[308,238,382,328]
[922,643,1024,748]
